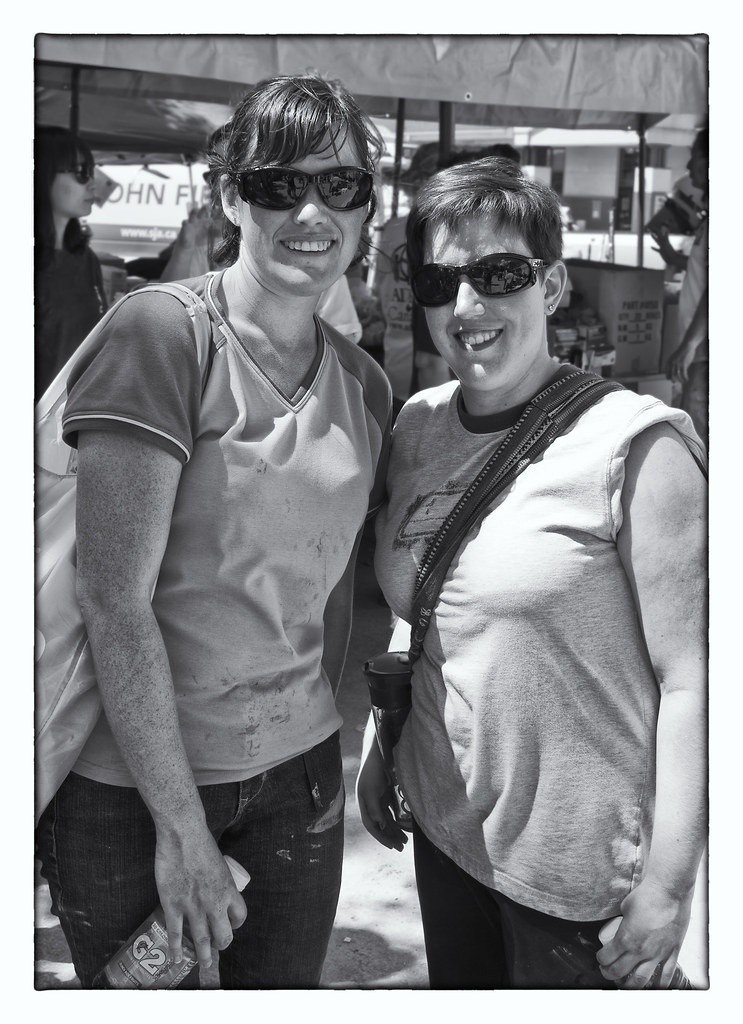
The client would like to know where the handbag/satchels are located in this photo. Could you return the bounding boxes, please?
[34,280,213,832]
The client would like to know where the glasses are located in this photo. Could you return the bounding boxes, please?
[230,164,376,211]
[202,171,214,186]
[56,162,97,185]
[409,251,548,307]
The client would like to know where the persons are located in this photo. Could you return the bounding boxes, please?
[33,74,396,990]
[34,125,707,444]
[355,156,707,990]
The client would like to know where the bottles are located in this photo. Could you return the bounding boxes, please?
[598,916,696,988]
[91,856,250,987]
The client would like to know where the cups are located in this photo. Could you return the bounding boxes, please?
[364,651,414,832]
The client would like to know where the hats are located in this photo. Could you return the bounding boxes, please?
[399,141,459,190]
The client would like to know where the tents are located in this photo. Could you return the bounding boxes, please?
[34,34,709,271]
